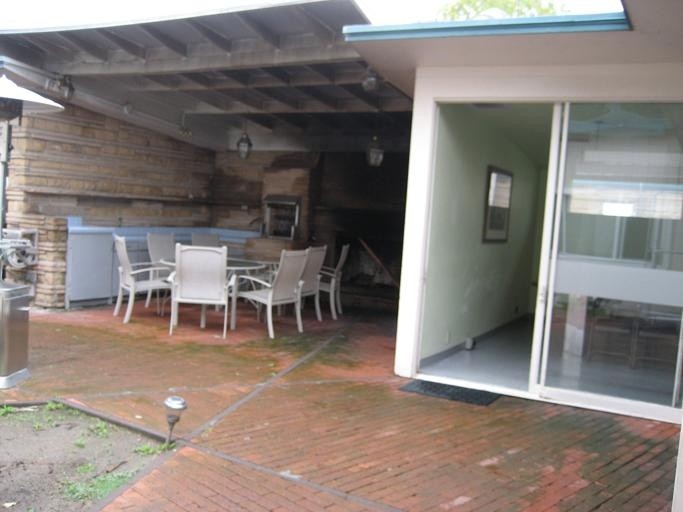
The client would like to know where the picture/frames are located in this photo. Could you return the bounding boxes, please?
[482,164,514,243]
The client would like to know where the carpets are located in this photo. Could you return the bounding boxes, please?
[398,380,502,407]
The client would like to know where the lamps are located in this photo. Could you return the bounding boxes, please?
[58,79,76,103]
[234,129,253,162]
[366,133,383,166]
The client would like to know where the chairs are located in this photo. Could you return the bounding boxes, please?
[112,229,350,340]
